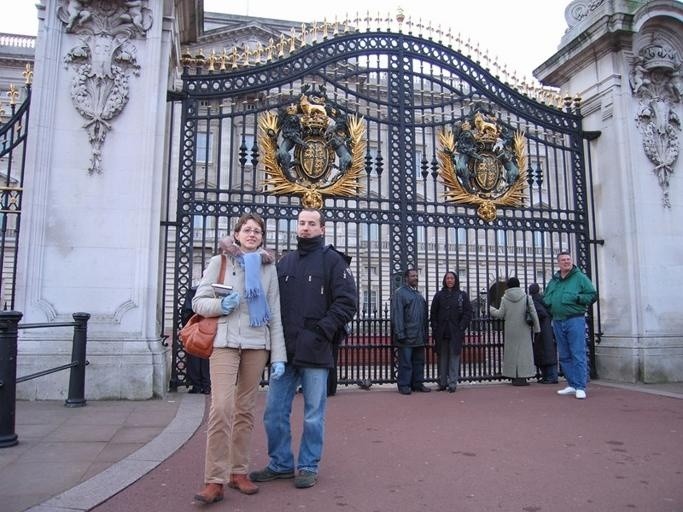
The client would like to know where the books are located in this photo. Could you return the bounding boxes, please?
[211,283,233,298]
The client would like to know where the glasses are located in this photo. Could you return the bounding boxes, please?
[240,228,263,237]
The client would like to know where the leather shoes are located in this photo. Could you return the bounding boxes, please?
[413,385,430,392]
[538,379,558,384]
[400,387,411,394]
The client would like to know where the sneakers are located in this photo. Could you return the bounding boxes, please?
[575,389,586,399]
[249,470,294,482]
[194,483,223,503]
[557,386,576,395]
[227,474,258,494]
[294,471,317,488]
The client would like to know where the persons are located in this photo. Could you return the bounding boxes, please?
[430,271,472,392]
[542,252,598,398]
[181,266,215,394]
[490,277,540,386]
[250,208,356,488]
[529,283,558,384]
[191,212,287,503]
[327,324,349,397]
[391,269,432,394]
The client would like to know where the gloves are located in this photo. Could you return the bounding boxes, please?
[270,362,286,380]
[221,292,239,313]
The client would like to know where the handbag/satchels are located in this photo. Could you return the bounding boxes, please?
[526,312,532,326]
[180,314,217,359]
